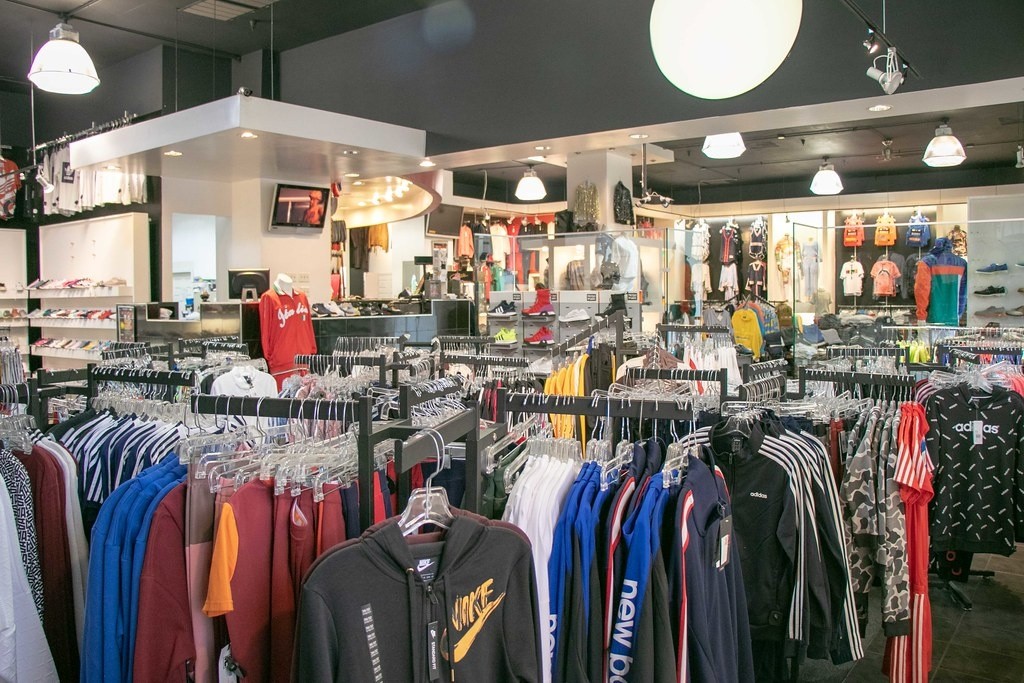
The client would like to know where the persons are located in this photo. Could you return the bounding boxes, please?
[479,252,501,304]
[259,274,318,392]
[611,230,638,292]
[302,188,325,224]
[595,223,614,283]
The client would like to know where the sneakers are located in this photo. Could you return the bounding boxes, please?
[487,300,516,318]
[974,286,1005,296]
[490,328,517,345]
[310,297,402,317]
[523,327,555,346]
[975,306,1006,317]
[975,262,1008,275]
[558,309,590,322]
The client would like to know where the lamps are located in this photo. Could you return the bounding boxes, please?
[731,216,738,227]
[785,213,791,224]
[480,212,542,226]
[920,118,968,169]
[862,32,878,53]
[701,132,747,159]
[866,47,903,94]
[859,209,866,222]
[911,206,918,220]
[27,11,102,95]
[674,218,683,227]
[900,61,909,86]
[514,164,547,201]
[809,156,844,195]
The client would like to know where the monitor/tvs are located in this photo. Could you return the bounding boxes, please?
[228,269,270,299]
[425,204,464,239]
[415,272,430,295]
[268,183,330,234]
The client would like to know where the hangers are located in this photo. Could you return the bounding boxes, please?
[26,112,139,161]
[882,254,888,261]
[849,254,855,263]
[916,258,920,265]
[9,296,1024,561]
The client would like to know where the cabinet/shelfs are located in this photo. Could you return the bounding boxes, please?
[29,212,151,400]
[523,290,560,363]
[599,289,643,348]
[489,289,524,376]
[0,229,31,378]
[560,290,599,356]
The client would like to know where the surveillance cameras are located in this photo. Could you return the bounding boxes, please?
[636,197,651,207]
[660,196,674,204]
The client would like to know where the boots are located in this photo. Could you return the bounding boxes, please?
[522,289,555,317]
[594,294,627,319]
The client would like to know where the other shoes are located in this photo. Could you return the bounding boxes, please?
[1018,288,1024,293]
[1006,306,1024,316]
[1015,263,1024,268]
[0,277,127,354]
